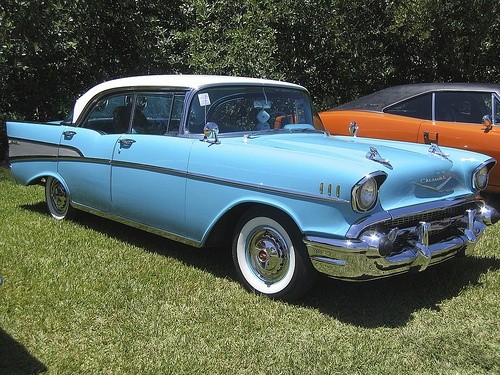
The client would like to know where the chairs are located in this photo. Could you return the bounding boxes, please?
[112,106,151,134]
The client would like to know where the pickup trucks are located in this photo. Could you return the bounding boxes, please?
[5,74,500,306]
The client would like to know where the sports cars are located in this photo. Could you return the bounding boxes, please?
[275,81,500,196]
